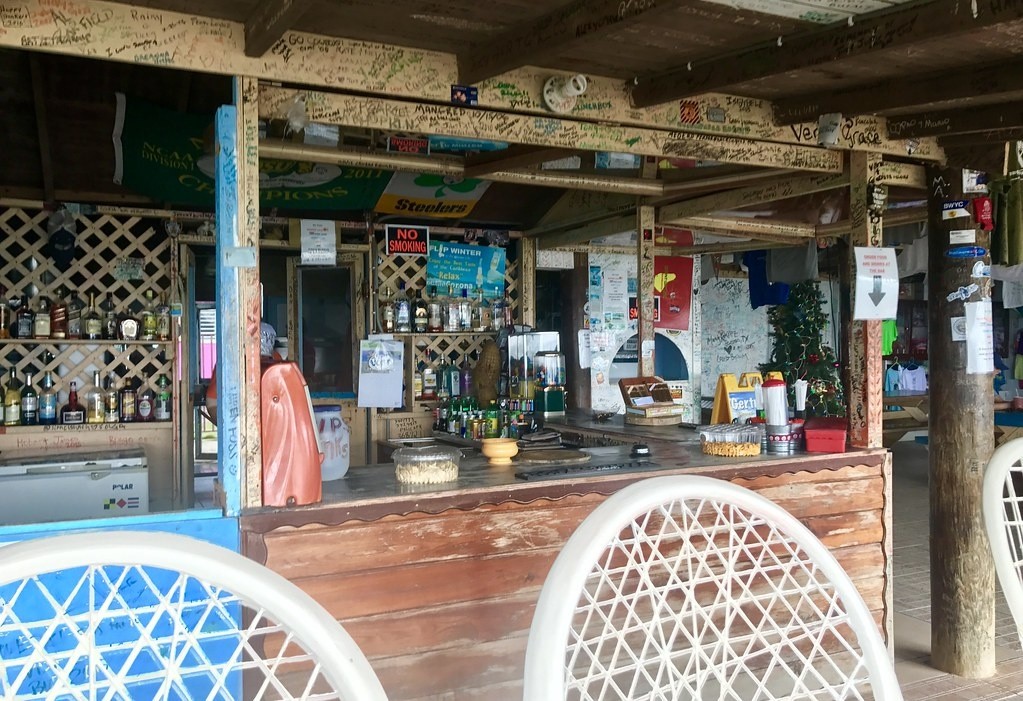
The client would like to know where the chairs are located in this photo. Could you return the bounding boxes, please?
[1,527,388,701]
[981,434,1023,668]
[522,473,904,701]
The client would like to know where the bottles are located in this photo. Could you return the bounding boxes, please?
[0,365,173,427]
[1,285,170,341]
[383,280,514,333]
[435,392,527,442]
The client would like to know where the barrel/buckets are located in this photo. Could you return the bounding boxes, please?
[313,404,350,483]
[750,421,804,454]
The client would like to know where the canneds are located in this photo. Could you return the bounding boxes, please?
[465,419,486,441]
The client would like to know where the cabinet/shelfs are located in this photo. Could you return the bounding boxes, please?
[383,330,500,414]
[1,335,175,432]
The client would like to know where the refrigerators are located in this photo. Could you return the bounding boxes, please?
[1,444,148,526]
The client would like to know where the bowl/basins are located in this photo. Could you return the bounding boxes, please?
[482,437,518,467]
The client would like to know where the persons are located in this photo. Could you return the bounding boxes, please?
[207,321,284,427]
[994,350,1013,409]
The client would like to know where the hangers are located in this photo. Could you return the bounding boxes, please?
[887,356,903,370]
[903,357,925,372]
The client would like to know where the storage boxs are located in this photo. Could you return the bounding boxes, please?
[804,416,848,453]
[532,351,567,386]
[695,424,763,457]
[508,331,561,398]
[391,445,463,485]
